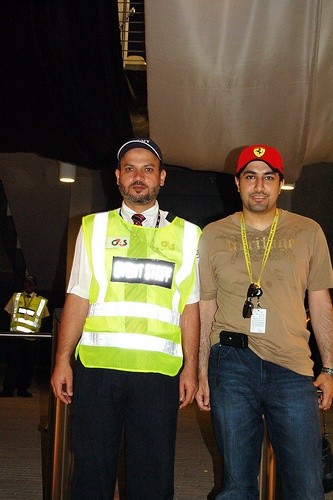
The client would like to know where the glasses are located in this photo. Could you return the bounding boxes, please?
[242,283,257,319]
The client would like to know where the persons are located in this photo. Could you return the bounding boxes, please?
[50,136,203,499]
[195,144,333,499]
[0,274,50,397]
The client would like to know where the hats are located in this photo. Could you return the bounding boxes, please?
[236,144,284,177]
[24,275,37,281]
[117,138,162,162]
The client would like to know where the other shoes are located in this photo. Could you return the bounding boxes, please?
[18,392,32,397]
[0,392,13,397]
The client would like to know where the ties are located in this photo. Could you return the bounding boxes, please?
[127,214,148,258]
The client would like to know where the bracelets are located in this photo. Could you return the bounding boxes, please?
[321,367,333,375]
